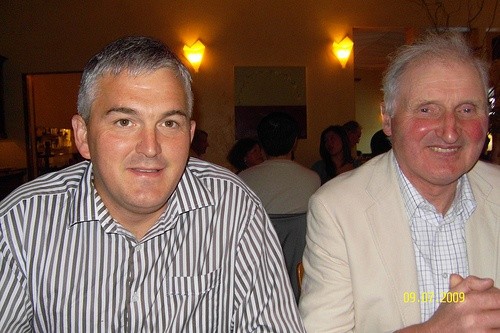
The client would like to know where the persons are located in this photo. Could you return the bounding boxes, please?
[299,27,500,333]
[0,33,307,333]
[189,119,393,215]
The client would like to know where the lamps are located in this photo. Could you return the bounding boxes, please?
[183,39,206,75]
[331,34,354,70]
